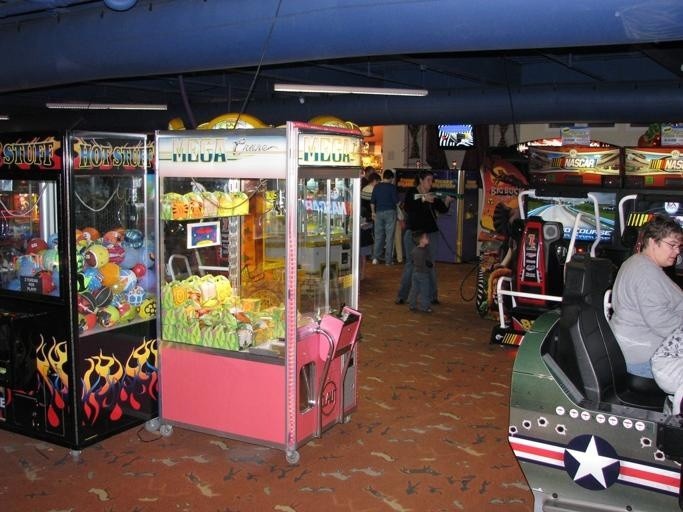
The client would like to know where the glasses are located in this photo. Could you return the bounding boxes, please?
[660,239,682,249]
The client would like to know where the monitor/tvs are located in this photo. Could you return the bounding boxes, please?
[435,121,477,151]
[519,187,620,248]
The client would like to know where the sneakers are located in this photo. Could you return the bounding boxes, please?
[385,260,396,267]
[372,259,378,264]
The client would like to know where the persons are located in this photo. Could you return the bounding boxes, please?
[370,169,402,266]
[360,166,375,189]
[609,213,683,378]
[395,168,454,307]
[409,231,438,315]
[360,172,382,240]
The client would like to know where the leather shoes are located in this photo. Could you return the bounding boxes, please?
[419,307,432,313]
[408,306,416,310]
[431,299,439,305]
[394,298,406,304]
